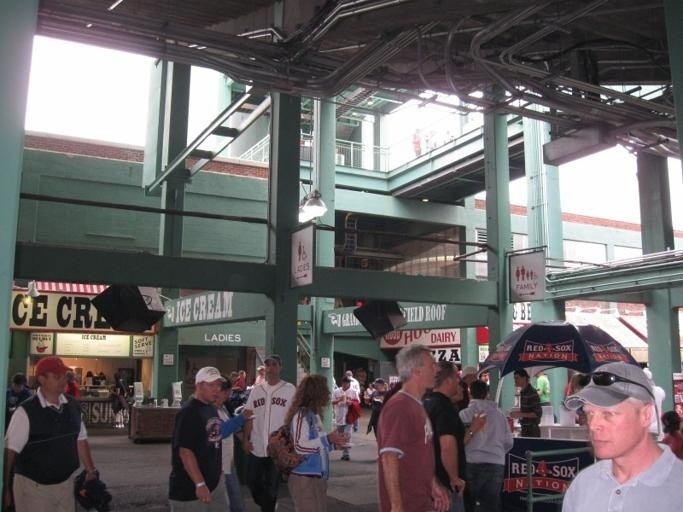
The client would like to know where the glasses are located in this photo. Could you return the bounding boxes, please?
[578,371,656,402]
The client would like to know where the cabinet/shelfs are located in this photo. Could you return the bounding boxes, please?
[129,404,182,444]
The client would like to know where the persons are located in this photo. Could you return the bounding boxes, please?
[242,354,296,512]
[561,362,683,512]
[332,370,389,460]
[64,370,130,431]
[224,366,266,484]
[376,341,514,512]
[275,374,350,512]
[660,411,683,461]
[5,373,32,436]
[2,356,99,512]
[209,375,255,512]
[168,366,231,512]
[512,369,542,439]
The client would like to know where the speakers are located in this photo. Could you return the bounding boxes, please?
[91,285,166,333]
[352,300,408,339]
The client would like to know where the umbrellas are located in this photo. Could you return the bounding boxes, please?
[484,319,640,384]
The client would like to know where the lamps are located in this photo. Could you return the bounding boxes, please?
[299,189,328,222]
[25,280,40,297]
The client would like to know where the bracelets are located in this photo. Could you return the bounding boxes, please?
[192,480,208,488]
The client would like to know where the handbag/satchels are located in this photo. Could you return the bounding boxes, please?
[75,470,111,510]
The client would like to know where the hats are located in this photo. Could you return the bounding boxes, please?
[195,366,227,385]
[264,354,282,366]
[564,361,656,410]
[12,373,28,388]
[460,365,477,379]
[33,357,73,376]
[372,378,385,385]
[342,375,352,382]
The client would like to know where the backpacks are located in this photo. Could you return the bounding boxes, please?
[267,424,306,473]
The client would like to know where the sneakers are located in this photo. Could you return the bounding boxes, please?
[341,455,349,460]
